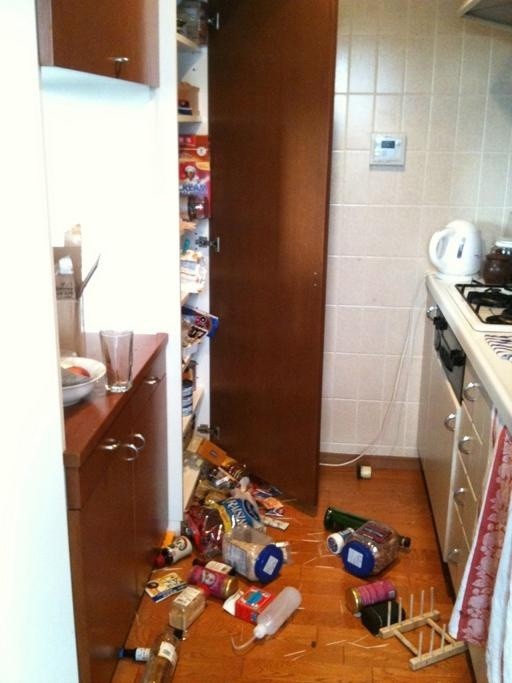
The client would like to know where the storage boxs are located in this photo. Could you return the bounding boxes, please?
[54,246,102,333]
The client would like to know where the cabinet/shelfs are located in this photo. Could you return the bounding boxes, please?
[178,1,340,523]
[63,332,169,682]
[36,1,159,88]
[415,272,512,682]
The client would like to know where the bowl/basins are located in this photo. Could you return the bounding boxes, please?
[62,357,106,407]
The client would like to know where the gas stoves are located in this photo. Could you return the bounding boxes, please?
[444,280,512,331]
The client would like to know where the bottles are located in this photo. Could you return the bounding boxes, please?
[323,506,411,549]
[345,577,397,612]
[192,557,233,574]
[179,193,208,220]
[253,587,304,639]
[156,536,200,568]
[118,645,154,663]
[343,519,400,578]
[186,564,238,598]
[136,625,181,682]
[221,525,284,584]
[166,582,212,630]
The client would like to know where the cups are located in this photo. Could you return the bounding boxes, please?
[101,329,133,393]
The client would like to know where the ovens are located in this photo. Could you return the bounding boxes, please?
[426,306,466,406]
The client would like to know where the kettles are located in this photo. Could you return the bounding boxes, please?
[429,220,481,281]
